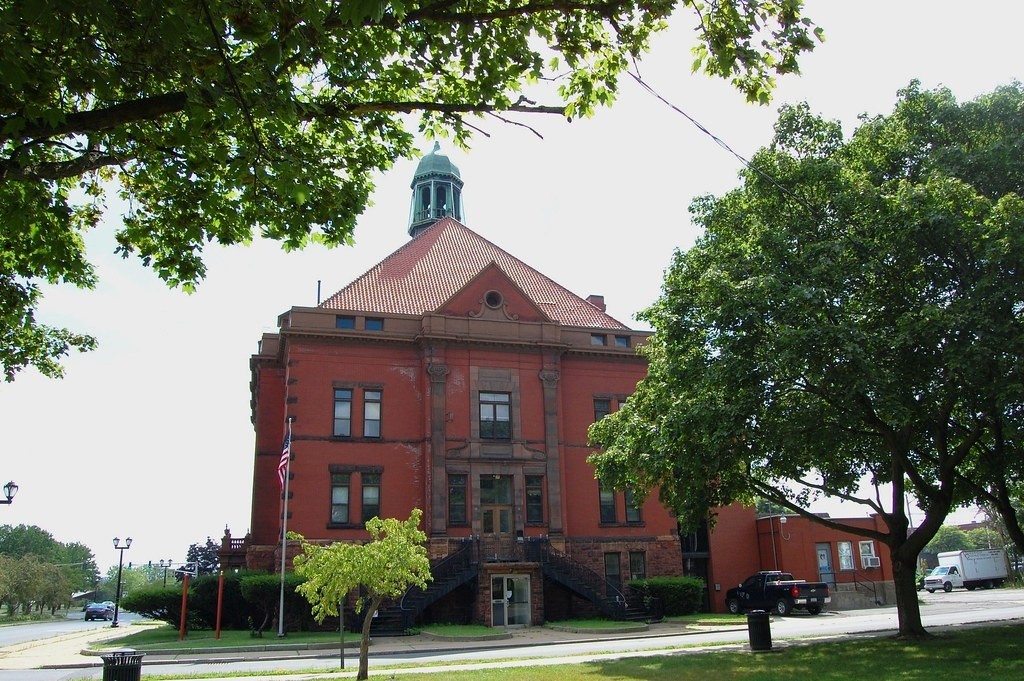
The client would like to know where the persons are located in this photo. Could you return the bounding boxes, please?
[275,428,293,492]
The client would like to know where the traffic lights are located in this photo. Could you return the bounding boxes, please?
[148,561,151,568]
[128,562,131,570]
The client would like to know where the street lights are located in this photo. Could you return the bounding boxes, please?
[160,559,173,588]
[111,537,133,628]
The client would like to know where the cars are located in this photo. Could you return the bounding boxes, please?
[85,603,113,621]
[102,601,114,610]
[83,602,96,612]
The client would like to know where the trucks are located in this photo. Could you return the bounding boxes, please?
[925,548,1008,593]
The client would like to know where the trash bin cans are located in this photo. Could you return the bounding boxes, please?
[748,609,773,650]
[100,651,146,681]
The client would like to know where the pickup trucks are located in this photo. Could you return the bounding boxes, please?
[724,570,829,616]
